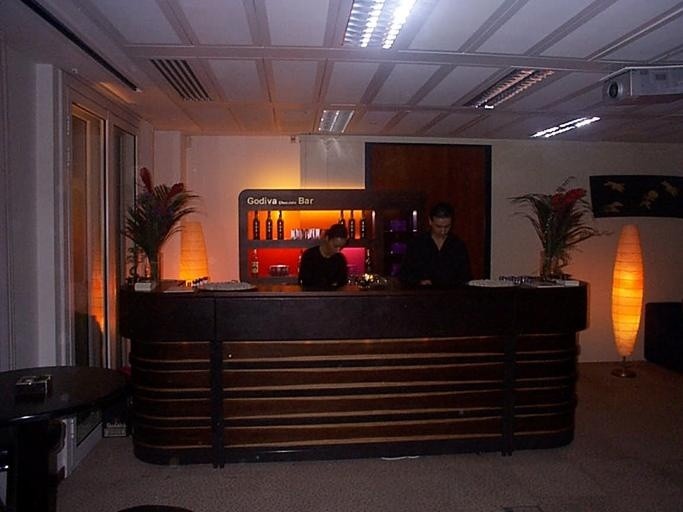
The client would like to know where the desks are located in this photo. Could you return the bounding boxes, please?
[0,363,133,512]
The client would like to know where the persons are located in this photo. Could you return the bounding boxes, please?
[400,202,471,287]
[298,224,348,290]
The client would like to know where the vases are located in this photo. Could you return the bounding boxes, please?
[538,250,573,282]
[140,255,162,285]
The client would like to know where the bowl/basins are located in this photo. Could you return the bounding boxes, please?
[269,264,288,276]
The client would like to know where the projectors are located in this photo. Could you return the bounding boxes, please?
[600,66,682,107]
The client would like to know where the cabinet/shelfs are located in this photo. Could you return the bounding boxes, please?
[238,190,427,285]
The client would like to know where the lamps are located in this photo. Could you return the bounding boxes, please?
[611,223,644,378]
[177,221,209,286]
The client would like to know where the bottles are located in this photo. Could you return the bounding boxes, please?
[296,254,301,274]
[276,211,285,240]
[252,210,260,239]
[347,209,357,239]
[337,210,345,225]
[360,210,368,239]
[265,211,273,241]
[364,249,377,273]
[251,248,259,277]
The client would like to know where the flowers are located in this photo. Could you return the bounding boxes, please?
[119,166,208,284]
[505,174,610,282]
